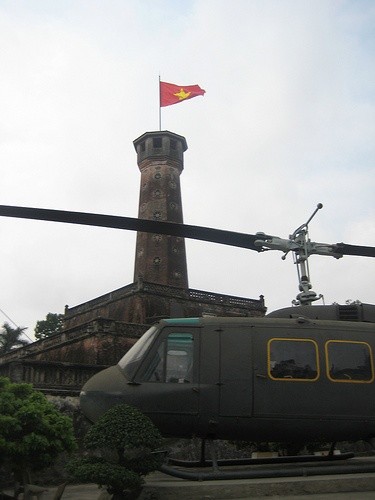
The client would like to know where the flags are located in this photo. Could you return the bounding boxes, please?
[160,81,206,107]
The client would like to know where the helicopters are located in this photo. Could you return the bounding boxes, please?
[0,203,375,481]
